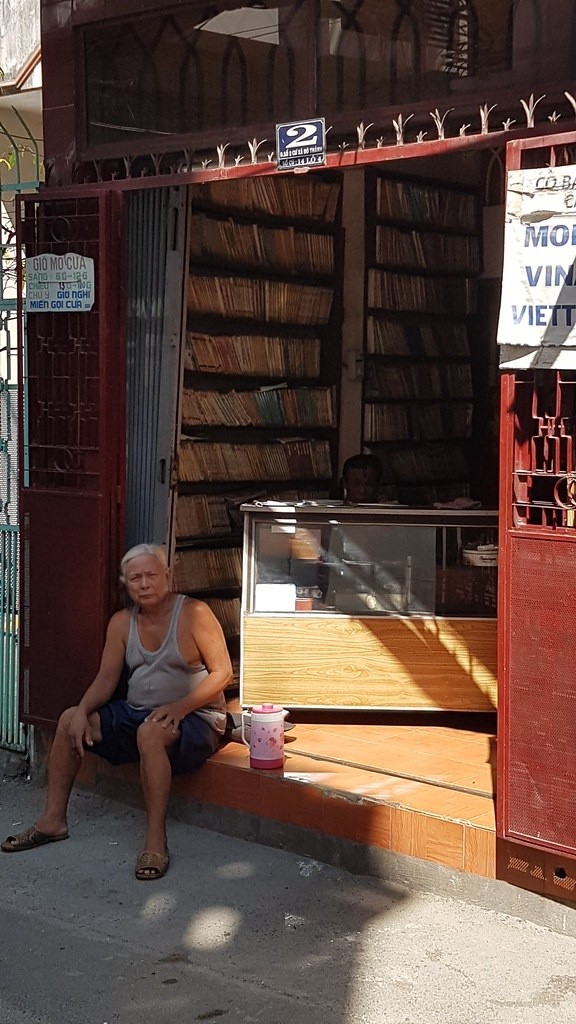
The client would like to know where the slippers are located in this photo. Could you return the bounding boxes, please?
[1,822,69,853]
[135,851,170,880]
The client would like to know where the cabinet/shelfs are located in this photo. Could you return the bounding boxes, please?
[239,499,500,715]
[171,170,345,694]
[340,164,485,504]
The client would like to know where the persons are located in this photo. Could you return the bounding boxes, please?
[0,544,234,880]
[342,454,384,503]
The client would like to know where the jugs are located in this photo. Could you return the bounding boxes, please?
[242,704,289,768]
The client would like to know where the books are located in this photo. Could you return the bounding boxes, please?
[366,176,473,506]
[171,163,342,678]
